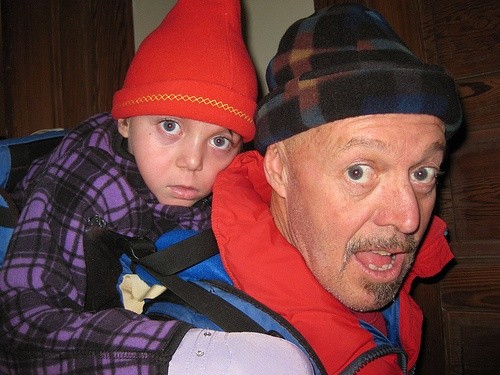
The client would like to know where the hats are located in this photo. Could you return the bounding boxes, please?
[254,1,462,156]
[112,1,257,144]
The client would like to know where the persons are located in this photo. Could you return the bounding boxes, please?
[0,1,317,375]
[110,1,462,374]
